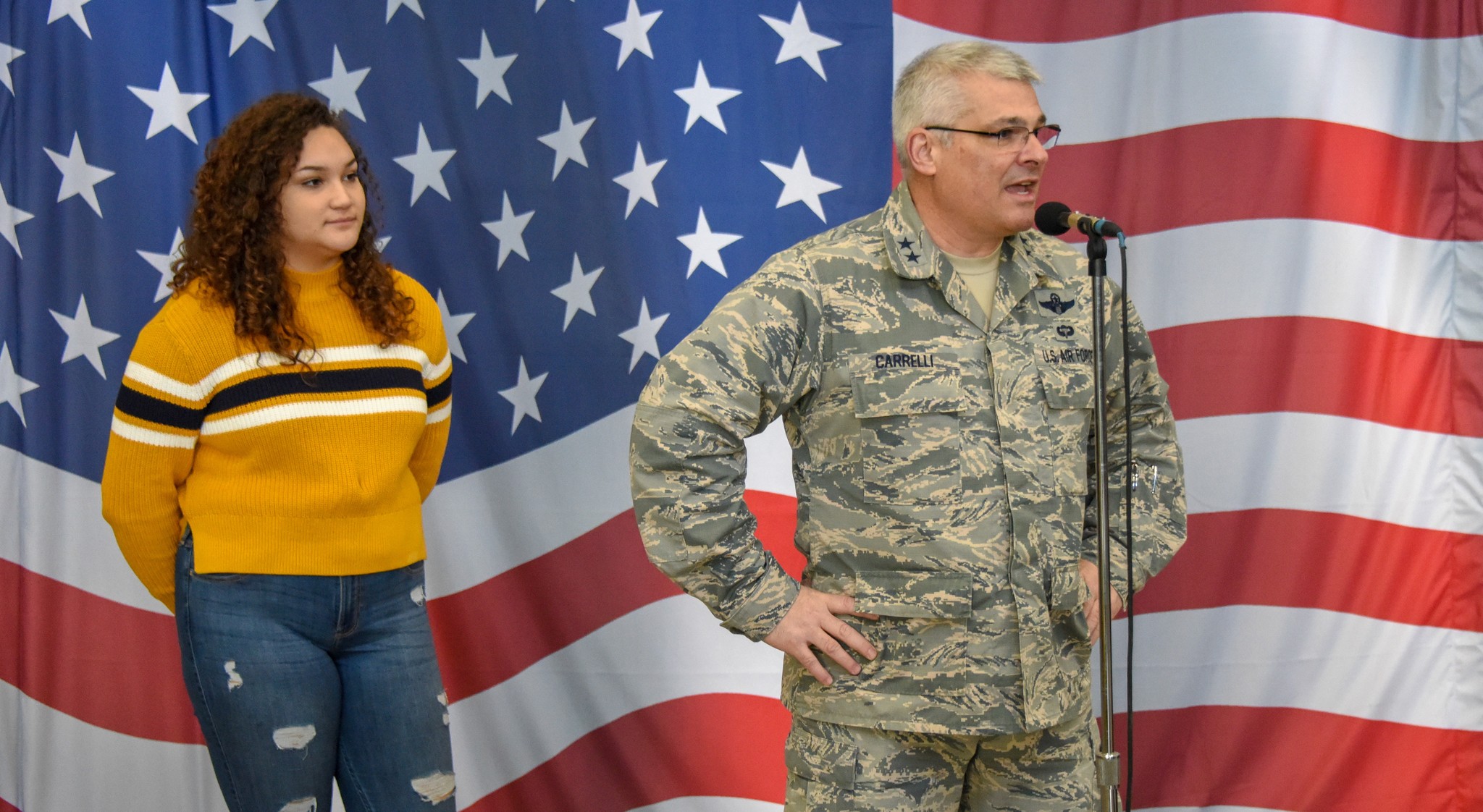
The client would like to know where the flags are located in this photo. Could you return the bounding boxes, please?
[0,0,1483,812]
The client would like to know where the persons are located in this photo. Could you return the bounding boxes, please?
[101,95,456,812]
[631,41,1189,812]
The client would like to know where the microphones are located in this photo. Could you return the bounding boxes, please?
[1034,202,1122,238]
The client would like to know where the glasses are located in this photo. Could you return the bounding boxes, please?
[925,124,1060,153]
